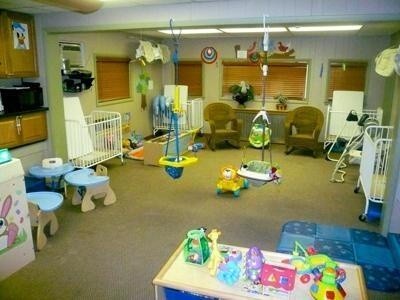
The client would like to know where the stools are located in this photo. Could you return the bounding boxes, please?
[25,161,117,251]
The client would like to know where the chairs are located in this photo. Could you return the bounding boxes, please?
[282,106,324,158]
[203,102,241,151]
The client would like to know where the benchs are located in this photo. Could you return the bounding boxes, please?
[143,129,191,167]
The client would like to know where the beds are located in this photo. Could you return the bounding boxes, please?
[323,103,395,223]
[64,110,124,169]
[152,98,205,138]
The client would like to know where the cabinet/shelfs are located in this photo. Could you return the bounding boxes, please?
[0,111,48,149]
[0,7,40,81]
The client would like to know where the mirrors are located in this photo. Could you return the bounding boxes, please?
[59,41,85,94]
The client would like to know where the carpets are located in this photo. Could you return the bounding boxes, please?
[276,220,400,293]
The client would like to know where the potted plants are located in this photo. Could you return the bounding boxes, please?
[276,95,288,110]
[228,80,255,108]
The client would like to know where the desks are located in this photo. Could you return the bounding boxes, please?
[0,157,35,282]
[230,108,294,145]
[152,239,368,300]
